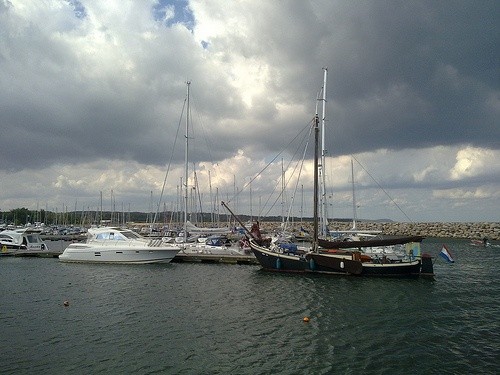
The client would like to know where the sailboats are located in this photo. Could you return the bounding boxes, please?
[221,65,436,279]
[0,80,385,265]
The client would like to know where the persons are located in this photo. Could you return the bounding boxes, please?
[245,221,272,250]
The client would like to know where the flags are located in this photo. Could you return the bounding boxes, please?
[439,243,457,264]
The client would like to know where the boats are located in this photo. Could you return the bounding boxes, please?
[0,227,50,256]
[58,227,182,264]
